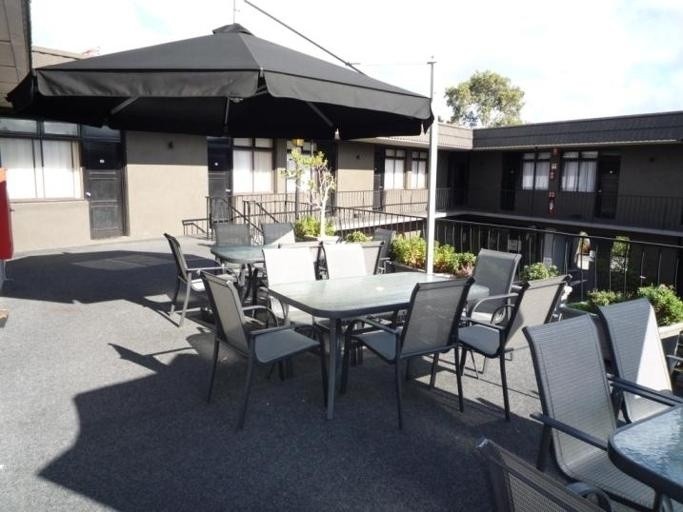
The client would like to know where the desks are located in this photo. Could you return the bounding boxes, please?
[609,402,682,512]
[263,268,489,421]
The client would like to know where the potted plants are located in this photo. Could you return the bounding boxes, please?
[559,283,683,378]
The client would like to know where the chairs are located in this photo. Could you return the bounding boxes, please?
[338,274,476,431]
[162,231,242,327]
[426,275,575,424]
[320,241,375,365]
[200,269,328,433]
[209,220,395,290]
[258,246,329,376]
[464,249,524,374]
[595,297,674,423]
[522,312,673,512]
[476,437,609,512]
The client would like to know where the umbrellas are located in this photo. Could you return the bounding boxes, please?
[1,22,432,152]
